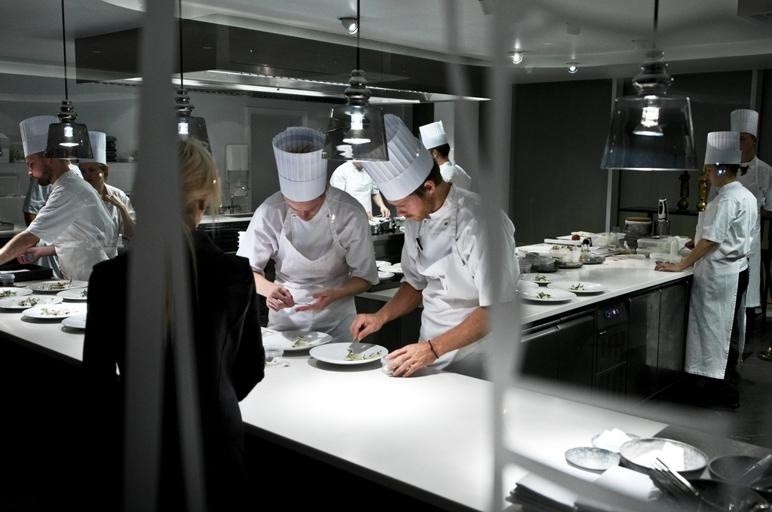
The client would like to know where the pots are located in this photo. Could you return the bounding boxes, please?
[370,217,406,234]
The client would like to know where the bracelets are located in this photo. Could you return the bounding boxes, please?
[427,339,441,360]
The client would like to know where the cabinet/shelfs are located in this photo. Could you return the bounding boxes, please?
[522,277,692,403]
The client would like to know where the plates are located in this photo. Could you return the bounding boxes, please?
[376,260,403,280]
[309,342,388,365]
[0,279,88,333]
[520,256,556,269]
[516,272,607,303]
[262,331,333,351]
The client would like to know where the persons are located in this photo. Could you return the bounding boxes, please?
[706,132,772,362]
[23,176,52,222]
[0,151,117,279]
[78,162,137,238]
[348,158,521,381]
[330,157,391,220]
[655,164,763,411]
[235,187,380,342]
[83,136,265,512]
[428,144,472,190]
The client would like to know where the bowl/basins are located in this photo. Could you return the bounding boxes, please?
[566,431,771,511]
[1,274,15,287]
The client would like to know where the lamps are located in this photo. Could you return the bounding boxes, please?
[567,62,579,73]
[509,49,524,64]
[322,0,389,161]
[173,0,211,153]
[43,0,93,160]
[600,1,700,174]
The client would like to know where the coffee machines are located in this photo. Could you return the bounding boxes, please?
[224,145,254,216]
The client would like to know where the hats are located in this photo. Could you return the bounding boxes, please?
[272,125,328,201]
[417,119,448,151]
[704,132,741,166]
[730,109,759,137]
[359,115,434,202]
[77,130,108,165]
[19,115,61,159]
